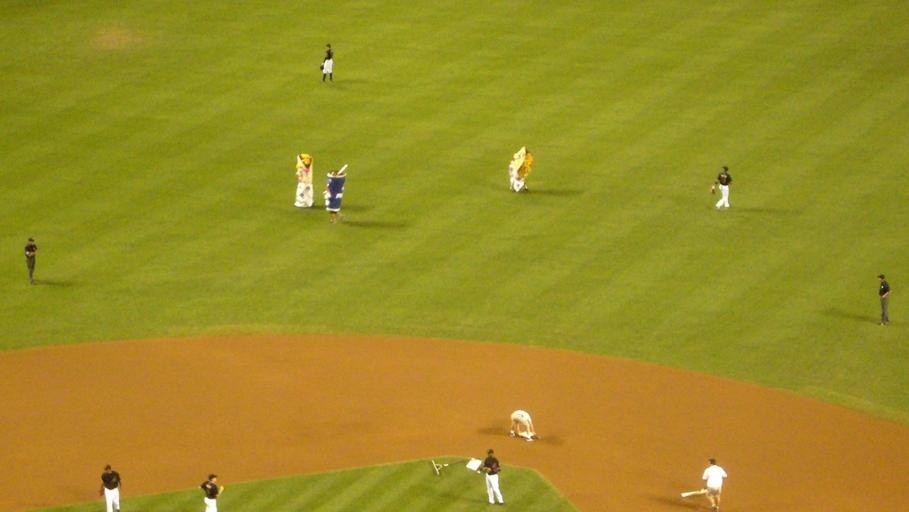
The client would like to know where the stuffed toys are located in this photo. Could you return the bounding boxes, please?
[294,153,314,208]
[324,164,348,225]
[508,146,534,192]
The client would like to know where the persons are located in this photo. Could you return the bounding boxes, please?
[199,474,224,512]
[320,44,334,82]
[100,464,122,512]
[509,410,536,442]
[479,449,504,505]
[702,459,727,512]
[877,274,891,326]
[715,166,732,209]
[24,238,38,283]
